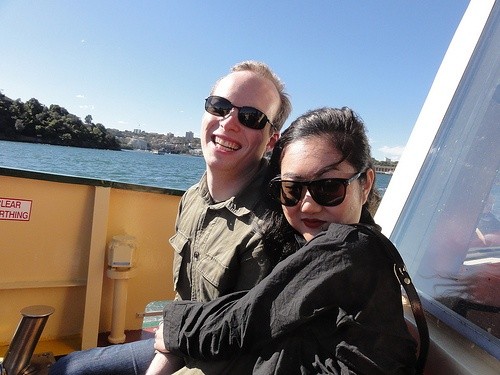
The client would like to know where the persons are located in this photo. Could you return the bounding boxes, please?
[47,61,418,375]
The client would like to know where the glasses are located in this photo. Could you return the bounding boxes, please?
[203,96,279,132]
[270,169,367,207]
